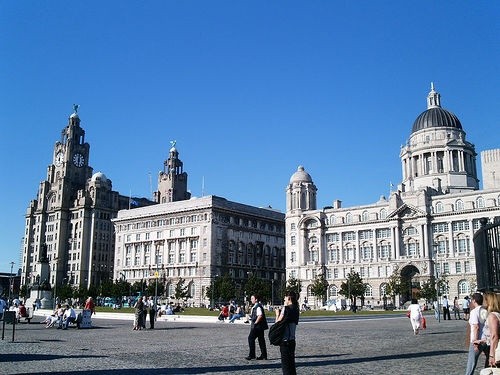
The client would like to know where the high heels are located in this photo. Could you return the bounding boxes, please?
[256,353,267,360]
[246,353,256,360]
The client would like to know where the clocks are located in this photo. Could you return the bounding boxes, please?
[72,152,85,168]
[56,152,63,166]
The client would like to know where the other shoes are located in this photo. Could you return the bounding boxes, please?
[244,321,250,323]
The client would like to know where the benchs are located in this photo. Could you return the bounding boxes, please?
[51,308,84,329]
[18,308,34,324]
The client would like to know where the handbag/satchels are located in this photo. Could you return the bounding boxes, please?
[473,338,486,352]
[422,317,426,329]
[406,312,410,318]
[268,320,291,347]
[218,314,223,320]
[480,364,500,375]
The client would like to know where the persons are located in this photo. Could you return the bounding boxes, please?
[349,305,357,312]
[157,302,181,315]
[133,296,155,330]
[463,296,471,321]
[433,297,439,320]
[214,304,219,309]
[245,294,269,360]
[275,290,299,375]
[465,293,488,375]
[0,295,6,319]
[454,297,461,320]
[220,303,242,322]
[85,297,96,315]
[423,304,426,310]
[13,302,26,317]
[443,296,452,320]
[40,303,76,329]
[128,298,131,306]
[473,292,500,368]
[408,298,423,334]
